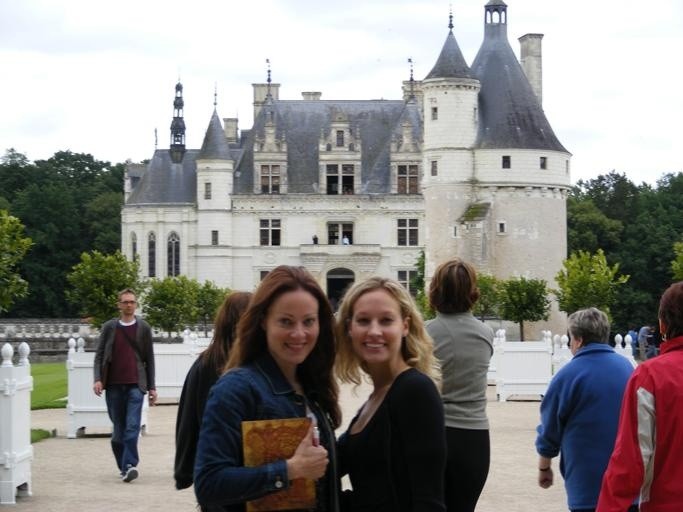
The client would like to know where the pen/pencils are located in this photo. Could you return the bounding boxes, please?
[313,427,320,482]
[150,396,153,400]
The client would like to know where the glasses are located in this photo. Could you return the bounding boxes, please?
[122,301,136,304]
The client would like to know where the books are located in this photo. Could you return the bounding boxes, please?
[239,417,316,512]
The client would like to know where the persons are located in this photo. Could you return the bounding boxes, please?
[193,265,348,512]
[628,326,656,363]
[175,293,252,490]
[93,288,157,482]
[534,309,639,512]
[423,259,494,512]
[342,235,350,245]
[331,276,448,511]
[313,234,319,244]
[596,281,683,512]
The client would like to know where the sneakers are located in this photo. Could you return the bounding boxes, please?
[122,465,139,482]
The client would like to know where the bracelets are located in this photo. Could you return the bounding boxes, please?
[149,388,155,390]
[538,467,550,471]
